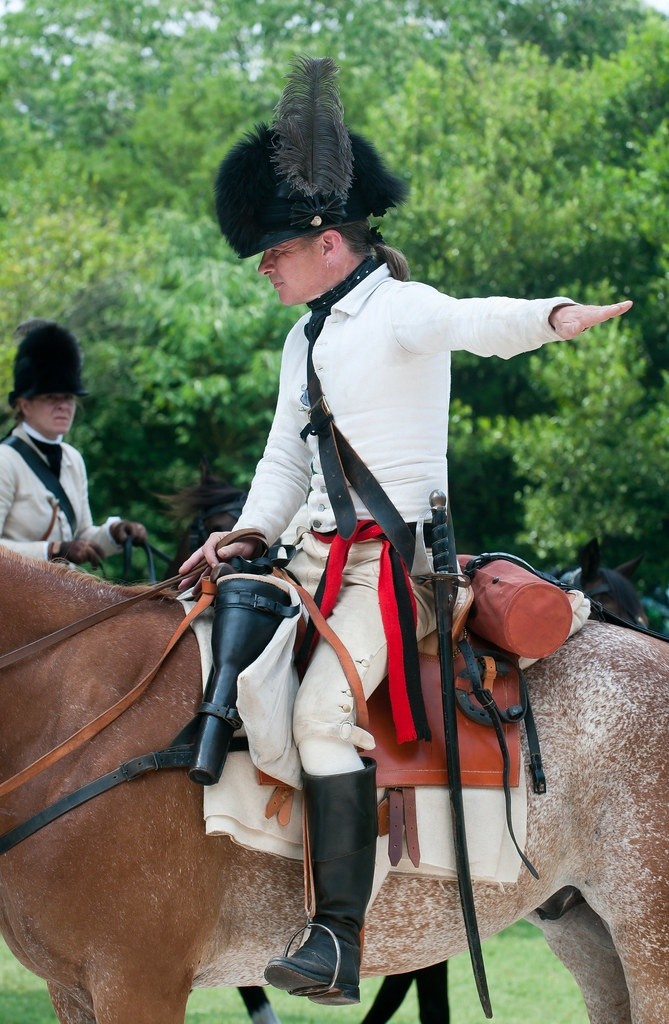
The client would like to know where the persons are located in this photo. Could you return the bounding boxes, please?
[0,323,148,571]
[178,119,633,1006]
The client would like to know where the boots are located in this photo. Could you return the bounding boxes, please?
[263,756,378,1004]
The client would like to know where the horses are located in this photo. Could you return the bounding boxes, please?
[0,459,669,1024]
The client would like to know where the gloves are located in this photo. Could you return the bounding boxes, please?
[110,520,147,547]
[48,541,106,572]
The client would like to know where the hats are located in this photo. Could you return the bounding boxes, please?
[15,321,84,397]
[214,55,406,258]
